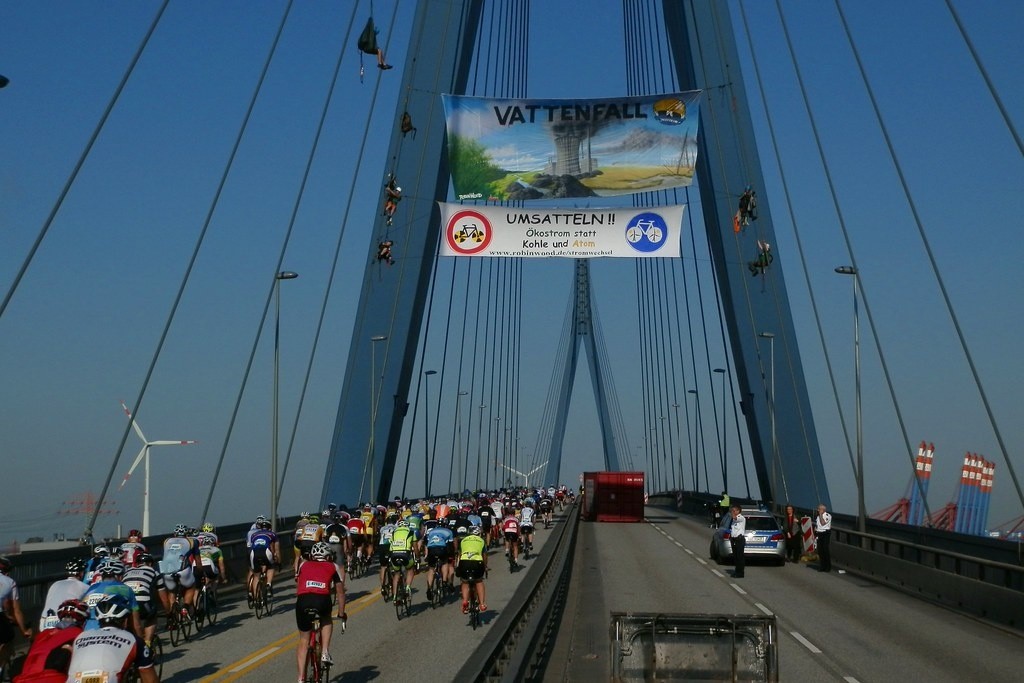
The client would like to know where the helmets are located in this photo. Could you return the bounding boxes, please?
[136,553,153,563]
[65,556,87,575]
[105,547,128,559]
[94,545,109,555]
[173,484,555,563]
[94,593,132,619]
[0,558,11,573]
[97,560,124,576]
[127,530,141,540]
[57,599,91,624]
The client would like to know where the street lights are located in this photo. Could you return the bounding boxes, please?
[494,417,502,490]
[688,389,699,494]
[271,270,299,548]
[370,335,390,507]
[456,390,468,498]
[759,333,779,511]
[834,265,871,549]
[672,405,683,491]
[424,369,437,498]
[474,405,487,492]
[714,368,729,493]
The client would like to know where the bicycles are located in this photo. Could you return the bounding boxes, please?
[137,599,164,683]
[347,541,372,581]
[543,508,553,531]
[382,552,424,622]
[0,621,31,683]
[427,555,454,609]
[302,607,348,683]
[506,535,520,575]
[168,573,228,647]
[463,570,485,631]
[522,529,533,561]
[248,562,278,619]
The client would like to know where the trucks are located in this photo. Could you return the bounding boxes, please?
[609,611,779,683]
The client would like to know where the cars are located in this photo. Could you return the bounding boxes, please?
[708,506,787,566]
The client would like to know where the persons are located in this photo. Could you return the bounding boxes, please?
[718,492,730,516]
[730,505,745,578]
[120,530,149,568]
[161,523,228,629]
[67,593,161,683]
[81,559,143,637]
[108,547,125,559]
[758,240,770,274]
[15,599,91,683]
[82,545,109,586]
[739,186,754,227]
[783,505,801,564]
[123,552,171,653]
[379,240,395,265]
[813,503,832,571]
[0,557,32,677]
[384,186,402,226]
[377,48,393,70]
[244,484,573,683]
[40,556,91,631]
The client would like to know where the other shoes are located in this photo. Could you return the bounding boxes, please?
[321,653,334,666]
[479,604,487,610]
[786,557,797,564]
[443,584,448,591]
[462,601,468,615]
[406,585,412,600]
[267,585,273,597]
[146,642,156,656]
[513,560,519,567]
[505,553,510,558]
[183,609,191,622]
[427,587,434,600]
[730,572,745,578]
[210,592,215,601]
[818,566,830,573]
